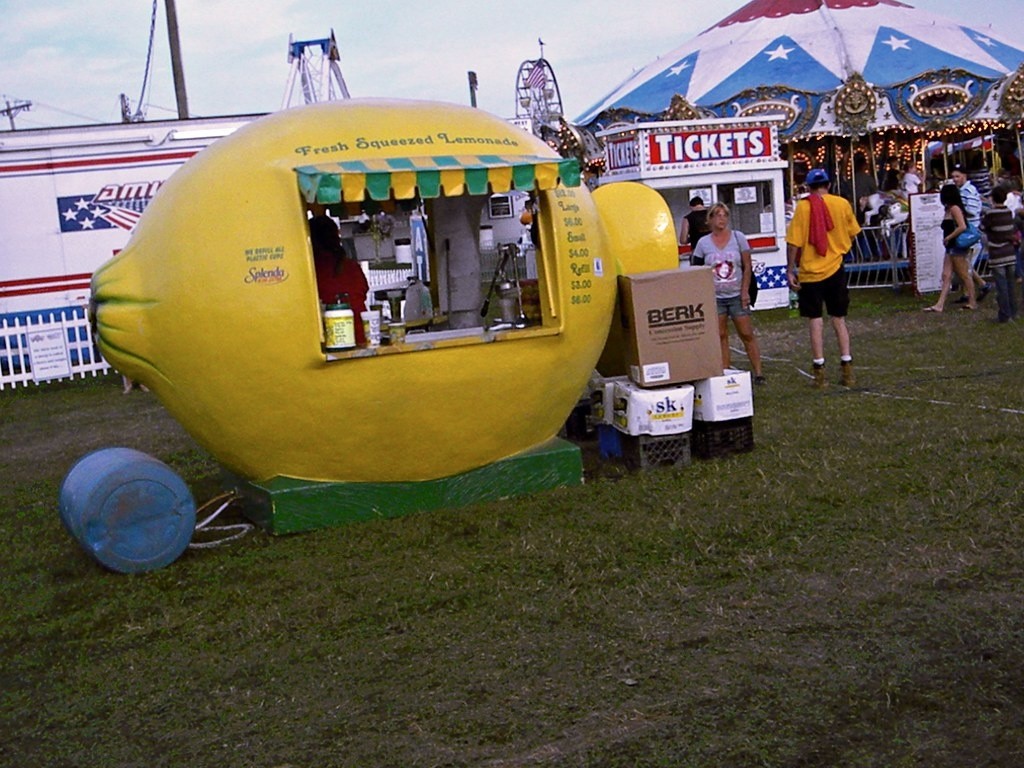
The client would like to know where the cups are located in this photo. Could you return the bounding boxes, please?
[389,323,406,347]
[360,309,382,350]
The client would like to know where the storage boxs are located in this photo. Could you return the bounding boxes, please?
[588,264,757,470]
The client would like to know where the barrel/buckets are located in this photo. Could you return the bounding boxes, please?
[59,447,195,576]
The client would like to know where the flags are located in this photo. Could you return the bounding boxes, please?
[525,59,546,90]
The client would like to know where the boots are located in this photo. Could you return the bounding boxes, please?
[838,359,858,390]
[810,361,825,388]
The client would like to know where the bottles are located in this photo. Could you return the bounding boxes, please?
[324,292,357,352]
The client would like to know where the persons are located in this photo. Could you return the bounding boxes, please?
[846,151,877,207]
[922,184,977,312]
[929,155,955,193]
[786,168,862,368]
[681,196,709,266]
[995,167,1024,283]
[877,156,903,197]
[691,202,767,385]
[952,166,994,303]
[969,156,996,209]
[307,214,369,350]
[976,187,1020,323]
[902,160,926,202]
[784,193,798,222]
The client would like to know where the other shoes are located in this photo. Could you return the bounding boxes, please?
[976,281,992,302]
[952,294,969,304]
[755,376,768,385]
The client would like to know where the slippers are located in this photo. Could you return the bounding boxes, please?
[923,306,935,312]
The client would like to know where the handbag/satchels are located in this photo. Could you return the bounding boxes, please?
[955,222,981,250]
[740,260,758,308]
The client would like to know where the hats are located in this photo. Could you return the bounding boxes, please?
[951,163,965,173]
[806,169,829,184]
